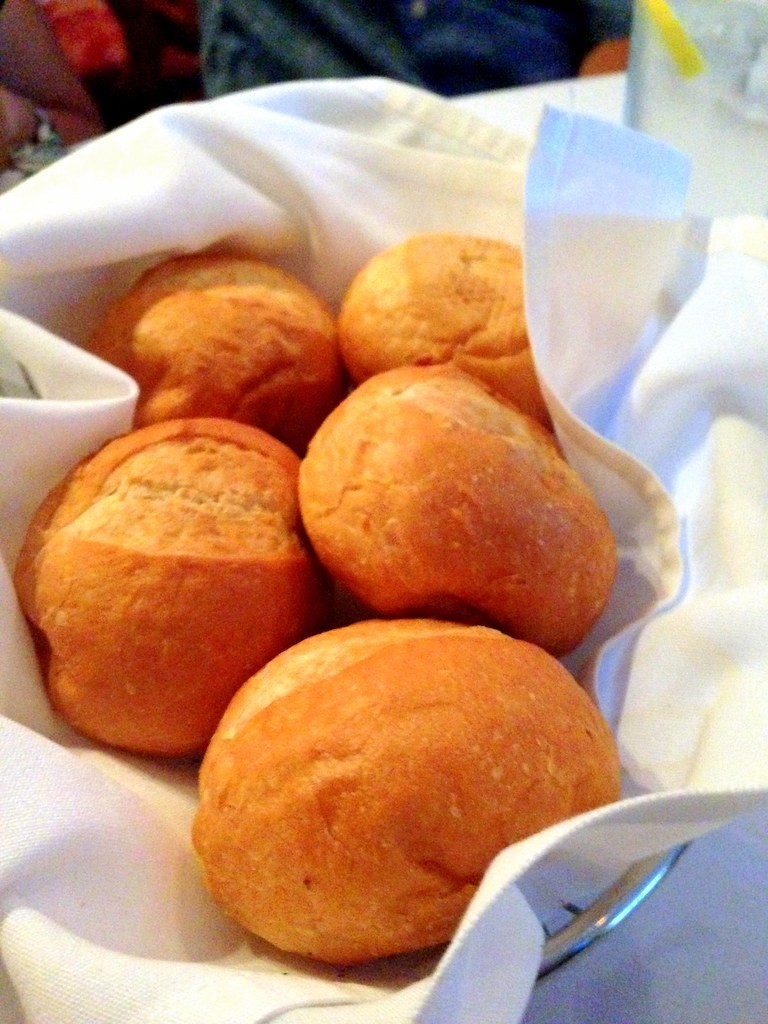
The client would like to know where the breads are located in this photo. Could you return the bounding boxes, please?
[86,248,337,444]
[14,415,320,754]
[297,364,615,654]
[190,619,620,963]
[339,232,546,424]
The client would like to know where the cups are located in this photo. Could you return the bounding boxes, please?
[624,1,767,221]
